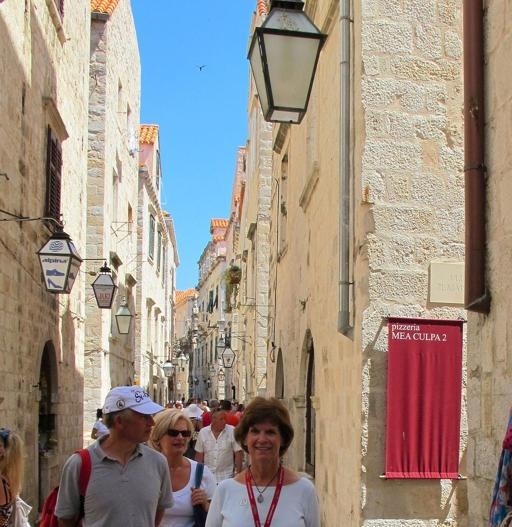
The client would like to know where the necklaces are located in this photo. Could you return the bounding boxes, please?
[247,464,283,503]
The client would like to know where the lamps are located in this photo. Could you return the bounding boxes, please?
[162,355,176,379]
[216,336,236,381]
[2,210,133,336]
[241,0,328,127]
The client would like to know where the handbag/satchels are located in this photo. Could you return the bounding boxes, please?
[193,463,207,527]
[14,497,32,527]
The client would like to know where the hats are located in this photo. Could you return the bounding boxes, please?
[180,403,205,418]
[102,385,165,415]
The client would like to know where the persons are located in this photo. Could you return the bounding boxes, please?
[148,403,218,526]
[1,426,36,526]
[53,384,178,526]
[203,396,323,526]
[91,407,110,439]
[166,396,248,483]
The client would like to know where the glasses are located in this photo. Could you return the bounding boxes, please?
[167,428,191,437]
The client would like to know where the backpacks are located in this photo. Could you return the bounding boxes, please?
[33,448,92,527]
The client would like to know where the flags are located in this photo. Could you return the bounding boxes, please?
[376,315,467,480]
